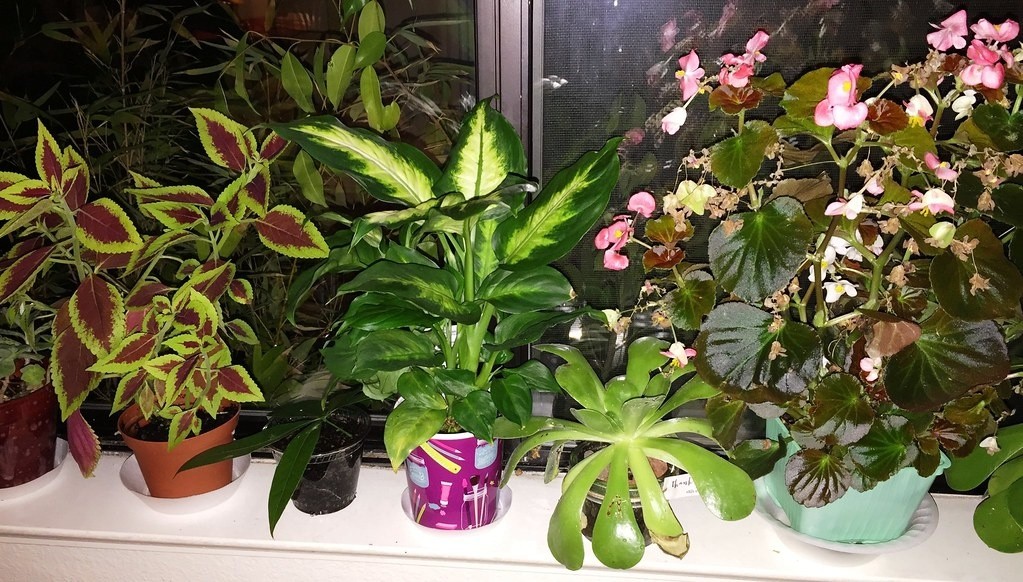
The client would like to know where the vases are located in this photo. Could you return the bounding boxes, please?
[765,418,953,543]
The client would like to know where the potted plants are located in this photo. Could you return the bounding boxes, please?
[243,94,624,530]
[0,106,398,539]
[501,336,756,571]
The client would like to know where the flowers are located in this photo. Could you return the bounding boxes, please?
[595,10,1023,507]
